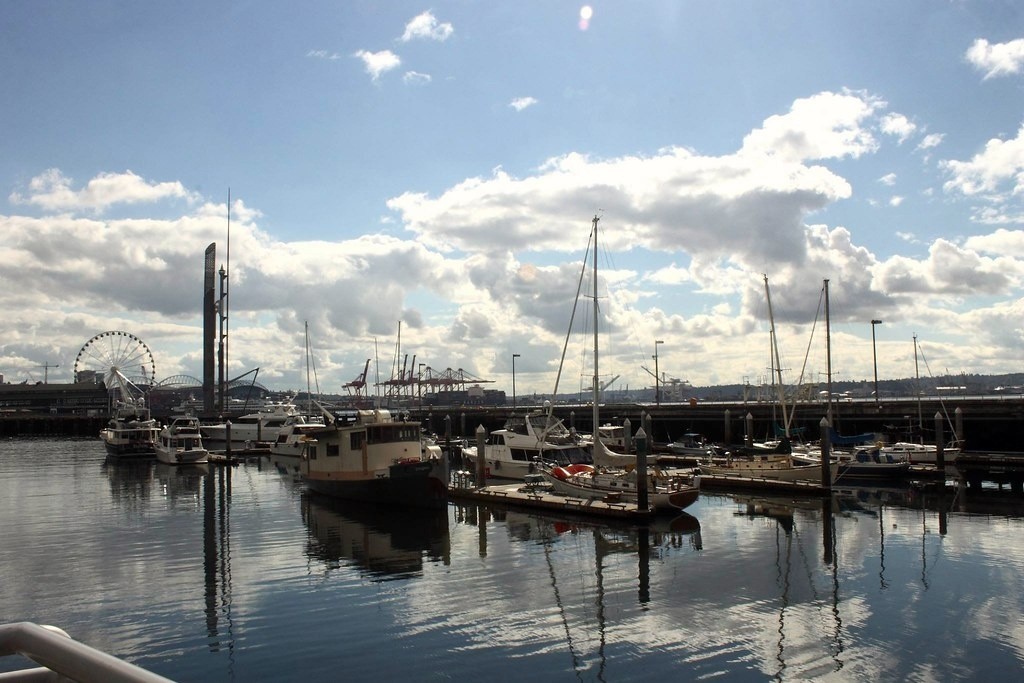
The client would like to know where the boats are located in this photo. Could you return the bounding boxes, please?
[99,396,159,458]
[460,412,606,481]
[169,398,274,419]
[156,414,207,464]
[300,483,450,584]
[297,409,441,499]
[688,438,844,488]
[667,432,725,458]
[199,401,304,441]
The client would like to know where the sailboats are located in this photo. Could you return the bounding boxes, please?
[758,273,964,471]
[269,321,349,457]
[531,208,699,518]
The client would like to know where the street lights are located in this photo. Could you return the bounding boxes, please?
[513,354,521,410]
[871,320,883,408]
[656,341,664,407]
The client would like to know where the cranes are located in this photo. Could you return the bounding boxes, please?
[341,353,497,406]
[641,365,689,402]
[585,375,621,402]
